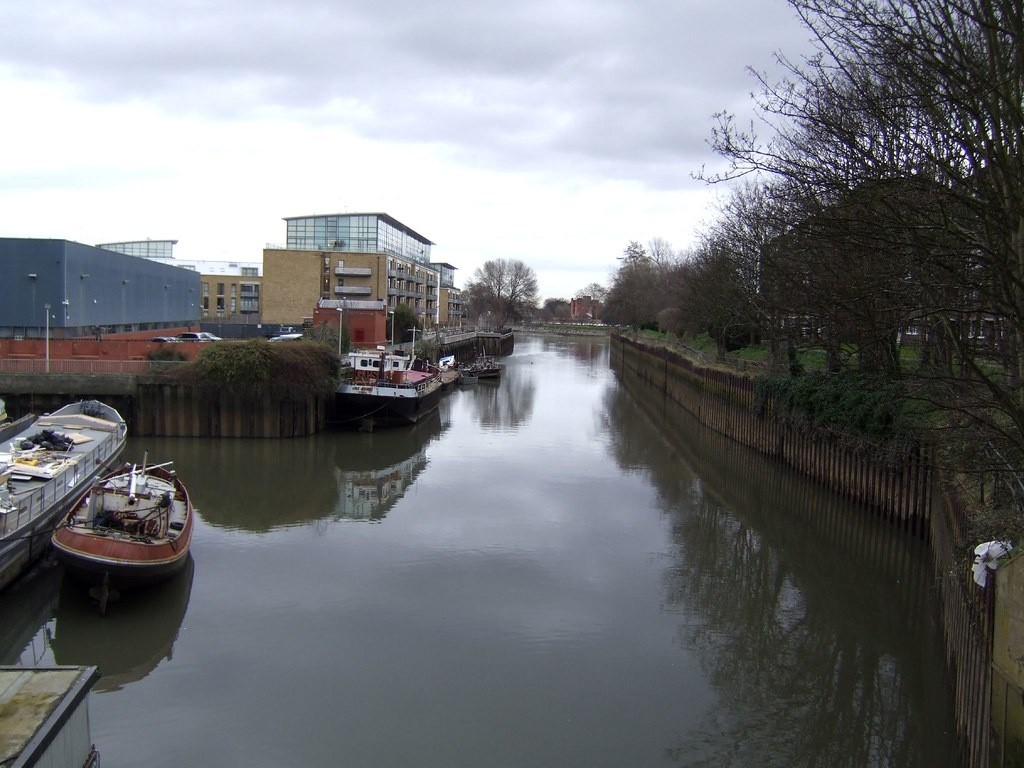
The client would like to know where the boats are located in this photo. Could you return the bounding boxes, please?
[433,354,461,391]
[468,345,503,379]
[334,344,441,433]
[0,398,128,593]
[460,366,479,385]
[51,449,195,614]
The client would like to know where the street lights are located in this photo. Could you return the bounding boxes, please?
[336,307,343,355]
[389,311,395,346]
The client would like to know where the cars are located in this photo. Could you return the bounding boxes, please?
[150,332,225,344]
